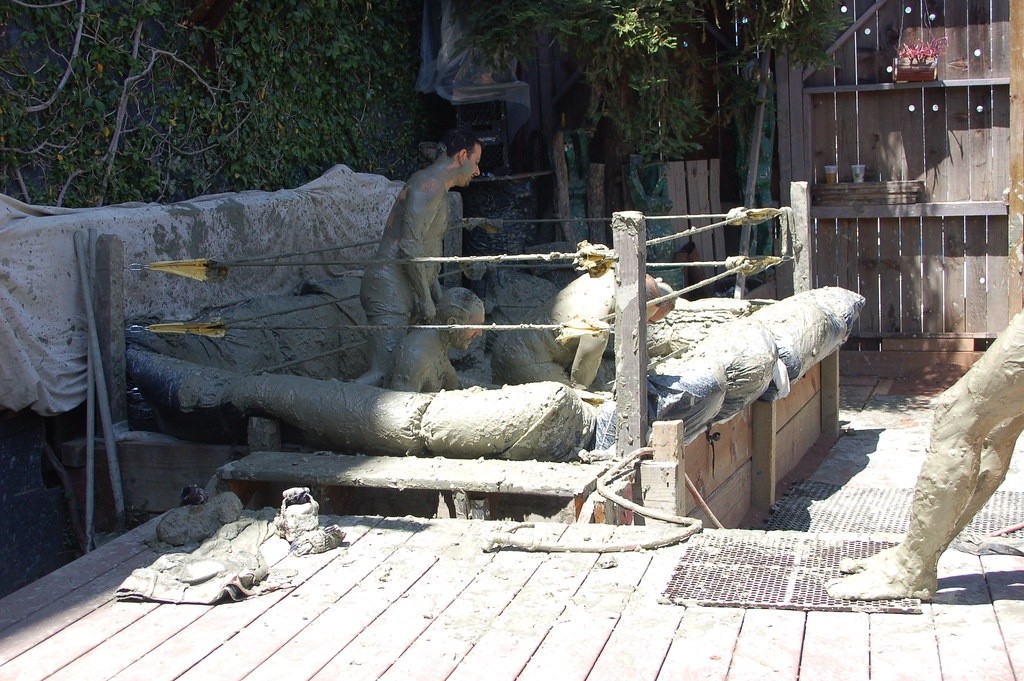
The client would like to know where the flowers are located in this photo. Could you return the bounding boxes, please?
[893,31,947,61]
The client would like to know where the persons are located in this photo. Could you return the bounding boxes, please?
[822,308,1023,604]
[351,130,482,388]
[490,264,675,391]
[380,288,485,392]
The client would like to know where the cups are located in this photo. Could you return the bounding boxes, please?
[851,165,865,184]
[824,166,838,185]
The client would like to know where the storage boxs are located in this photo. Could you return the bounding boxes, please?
[440,87,511,176]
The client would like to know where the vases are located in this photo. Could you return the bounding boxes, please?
[892,58,938,81]
